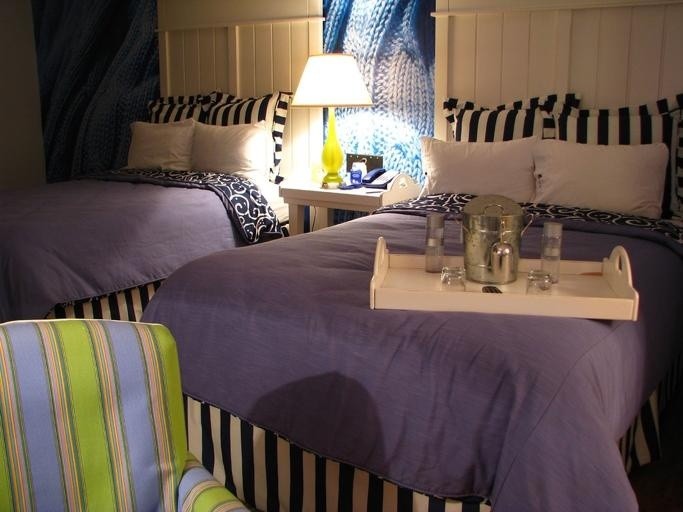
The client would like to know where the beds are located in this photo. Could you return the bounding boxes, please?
[0,167,286,324]
[139,194,682,512]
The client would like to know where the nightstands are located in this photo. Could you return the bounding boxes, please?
[279,173,420,237]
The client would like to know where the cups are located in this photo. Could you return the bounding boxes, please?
[525,269,553,296]
[440,266,465,293]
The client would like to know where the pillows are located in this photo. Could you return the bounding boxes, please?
[128,91,293,183]
[419,90,682,222]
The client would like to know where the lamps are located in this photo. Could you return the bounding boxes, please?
[291,54,373,189]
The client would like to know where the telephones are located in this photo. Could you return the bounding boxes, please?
[362,167,400,188]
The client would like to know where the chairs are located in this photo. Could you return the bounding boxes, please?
[0,318,252,512]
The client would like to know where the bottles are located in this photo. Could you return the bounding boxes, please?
[541,221,564,283]
[421,213,446,272]
